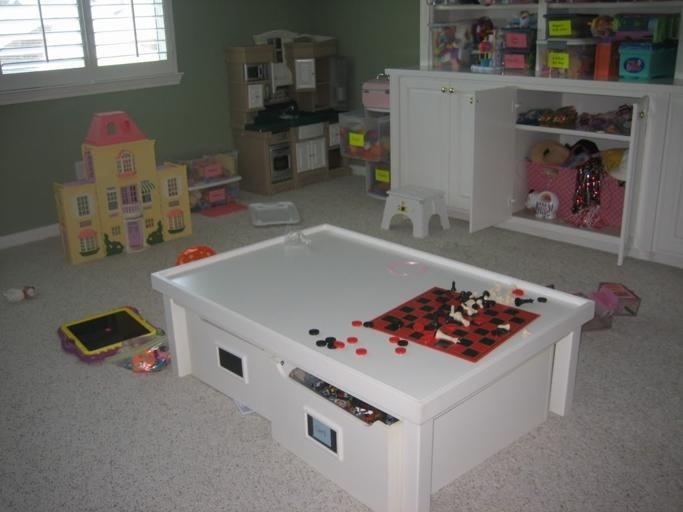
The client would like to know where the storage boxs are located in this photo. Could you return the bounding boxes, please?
[224,33,352,196]
[338,109,389,200]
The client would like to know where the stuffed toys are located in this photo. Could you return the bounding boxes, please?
[529,141,629,185]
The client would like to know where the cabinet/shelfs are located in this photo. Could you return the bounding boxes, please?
[384,67,503,227]
[649,82,681,273]
[419,1,682,85]
[469,75,669,270]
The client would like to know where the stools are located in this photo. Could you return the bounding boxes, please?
[382,185,451,237]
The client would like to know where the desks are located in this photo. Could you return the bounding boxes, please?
[150,224,596,512]
